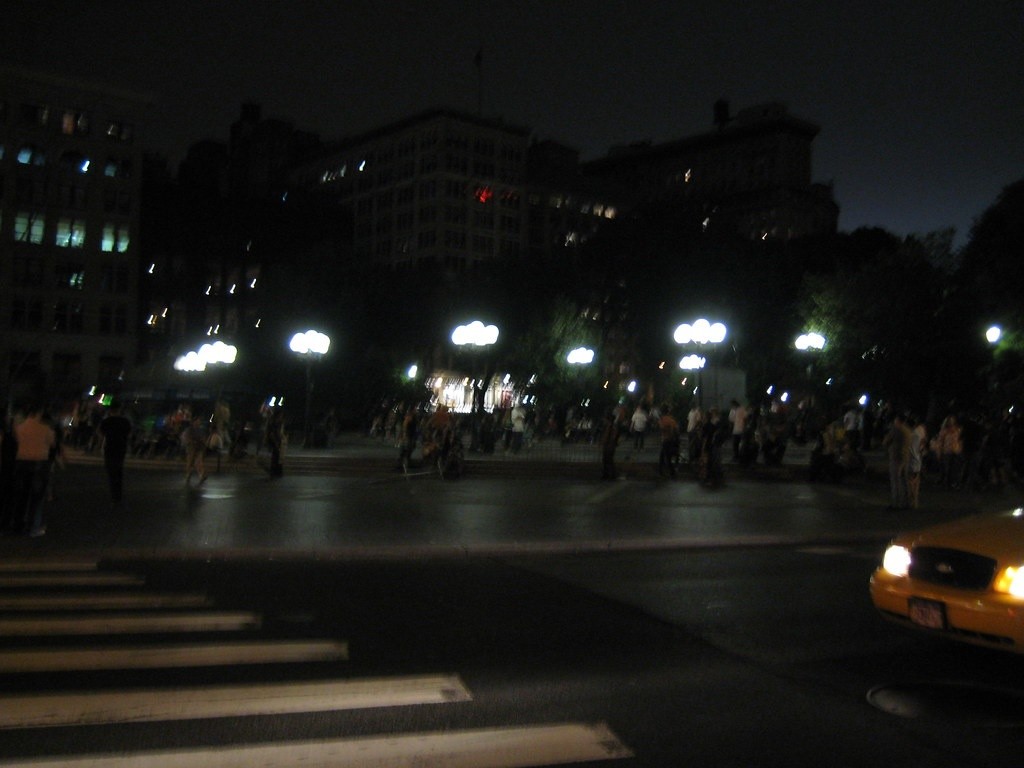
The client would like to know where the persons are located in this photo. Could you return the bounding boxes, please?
[2,392,335,536]
[372,389,1022,509]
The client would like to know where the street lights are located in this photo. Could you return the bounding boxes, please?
[289,329,331,450]
[451,320,501,453]
[673,317,727,407]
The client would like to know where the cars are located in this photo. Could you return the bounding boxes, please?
[868,492,1024,660]
